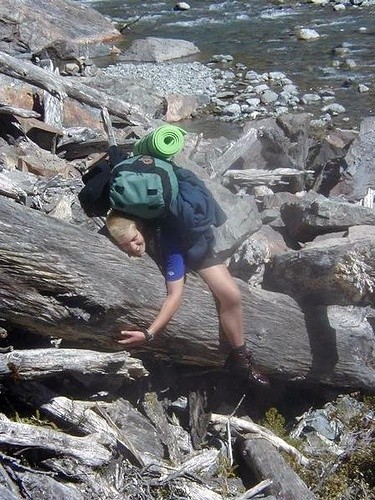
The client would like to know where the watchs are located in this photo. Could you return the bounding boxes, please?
[142,328,155,344]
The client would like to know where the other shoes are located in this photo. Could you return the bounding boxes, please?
[224,346,271,390]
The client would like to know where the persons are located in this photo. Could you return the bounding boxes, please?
[104,154,271,394]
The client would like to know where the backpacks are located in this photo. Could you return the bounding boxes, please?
[107,154,181,220]
